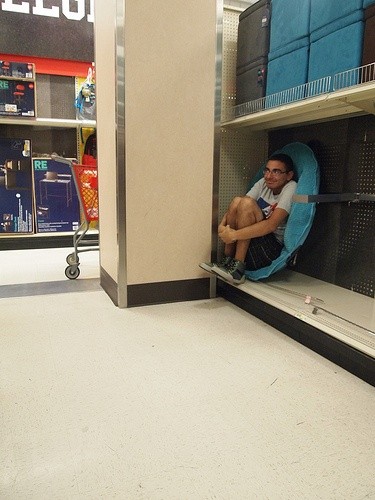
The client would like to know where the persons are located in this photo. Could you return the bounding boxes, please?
[198,153,298,285]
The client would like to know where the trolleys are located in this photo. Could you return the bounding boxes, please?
[49,151,100,280]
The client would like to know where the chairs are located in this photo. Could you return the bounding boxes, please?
[224,140,320,283]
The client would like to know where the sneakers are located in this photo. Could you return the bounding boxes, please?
[211,259,246,284]
[199,256,234,276]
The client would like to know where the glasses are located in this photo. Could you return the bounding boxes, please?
[262,167,288,176]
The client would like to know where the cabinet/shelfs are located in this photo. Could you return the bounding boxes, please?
[218,1,374,384]
[0,54,98,243]
